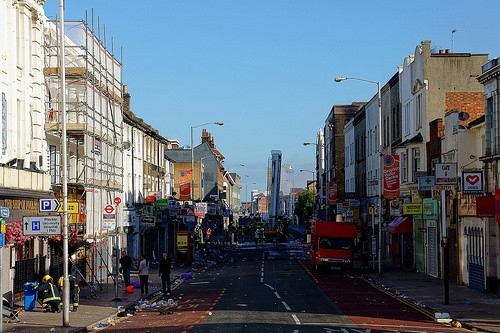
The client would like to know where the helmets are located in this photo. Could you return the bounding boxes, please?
[261,228,264,230]
[42,275,53,283]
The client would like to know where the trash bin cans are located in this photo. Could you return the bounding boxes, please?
[23,282,39,310]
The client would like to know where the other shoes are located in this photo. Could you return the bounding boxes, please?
[73,307,78,312]
[42,309,50,313]
[57,305,61,313]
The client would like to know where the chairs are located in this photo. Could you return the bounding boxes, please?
[2,291,23,323]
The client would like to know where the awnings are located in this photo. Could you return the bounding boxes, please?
[388,216,413,233]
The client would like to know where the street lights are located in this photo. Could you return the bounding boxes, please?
[191,121,224,201]
[333,76,383,276]
[300,169,315,221]
[200,155,221,206]
[303,142,328,221]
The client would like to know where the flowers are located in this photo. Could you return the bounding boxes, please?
[4,222,31,246]
[53,228,78,246]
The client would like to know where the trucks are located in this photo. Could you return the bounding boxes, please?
[309,221,357,272]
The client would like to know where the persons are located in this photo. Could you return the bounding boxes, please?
[158,252,171,296]
[58,275,81,312]
[254,228,265,246]
[120,251,131,287]
[30,275,63,313]
[138,254,150,295]
